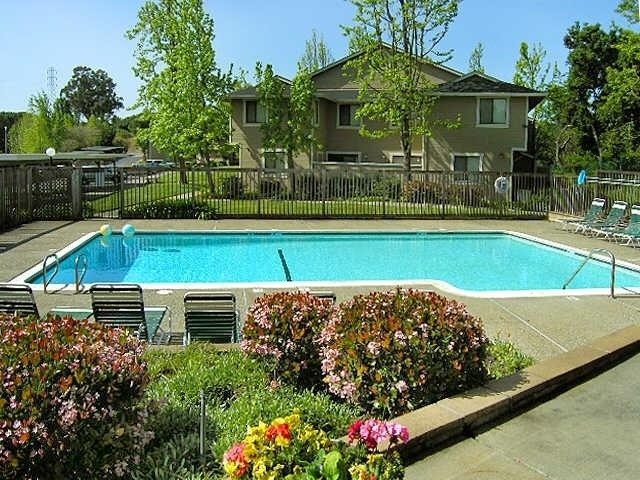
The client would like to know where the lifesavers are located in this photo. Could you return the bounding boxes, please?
[494,177,509,193]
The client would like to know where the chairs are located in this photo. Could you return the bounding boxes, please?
[552,197,640,248]
[0,283,93,319]
[183,292,240,343]
[90,282,172,345]
[312,291,336,305]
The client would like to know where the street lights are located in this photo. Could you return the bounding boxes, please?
[47,148,55,165]
[4,126,7,154]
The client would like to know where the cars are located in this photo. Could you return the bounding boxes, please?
[81,166,127,184]
[132,159,175,167]
